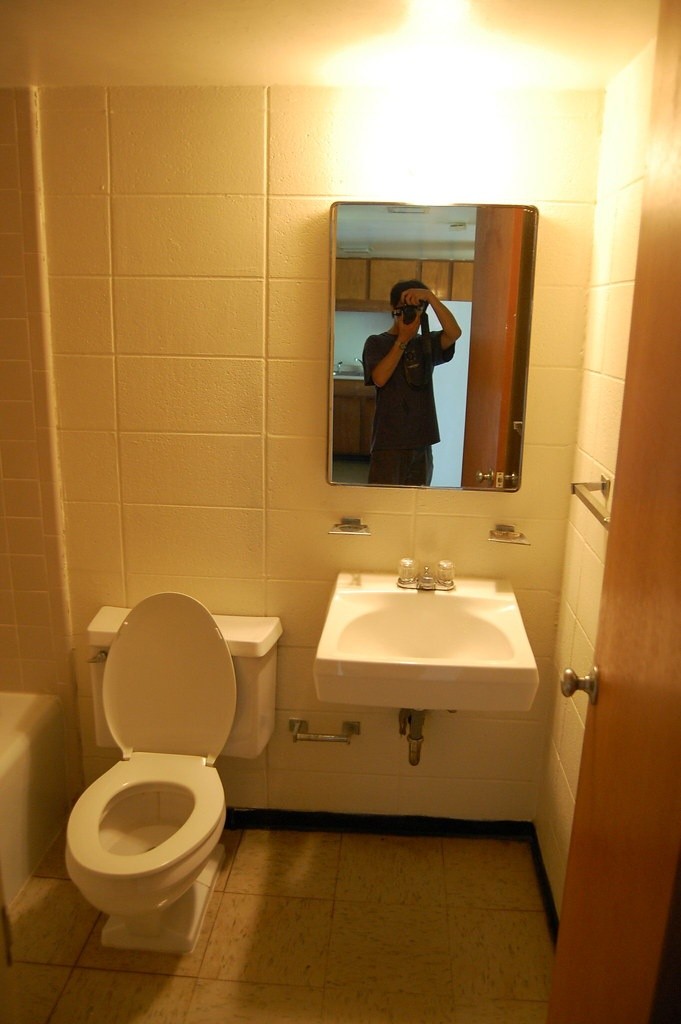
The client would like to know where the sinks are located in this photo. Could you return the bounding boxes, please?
[310,569,540,716]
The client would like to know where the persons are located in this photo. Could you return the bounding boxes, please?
[362,279,462,487]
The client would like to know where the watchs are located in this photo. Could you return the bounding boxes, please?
[394,340,407,350]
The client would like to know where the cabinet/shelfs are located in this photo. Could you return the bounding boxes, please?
[332,395,375,462]
[334,257,473,313]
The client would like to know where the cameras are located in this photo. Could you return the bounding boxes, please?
[399,297,418,325]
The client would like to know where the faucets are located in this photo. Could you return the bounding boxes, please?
[416,565,438,590]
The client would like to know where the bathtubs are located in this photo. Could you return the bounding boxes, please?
[0,687,63,909]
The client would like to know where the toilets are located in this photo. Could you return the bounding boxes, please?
[62,592,288,958]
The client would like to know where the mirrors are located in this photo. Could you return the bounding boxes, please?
[326,200,540,492]
